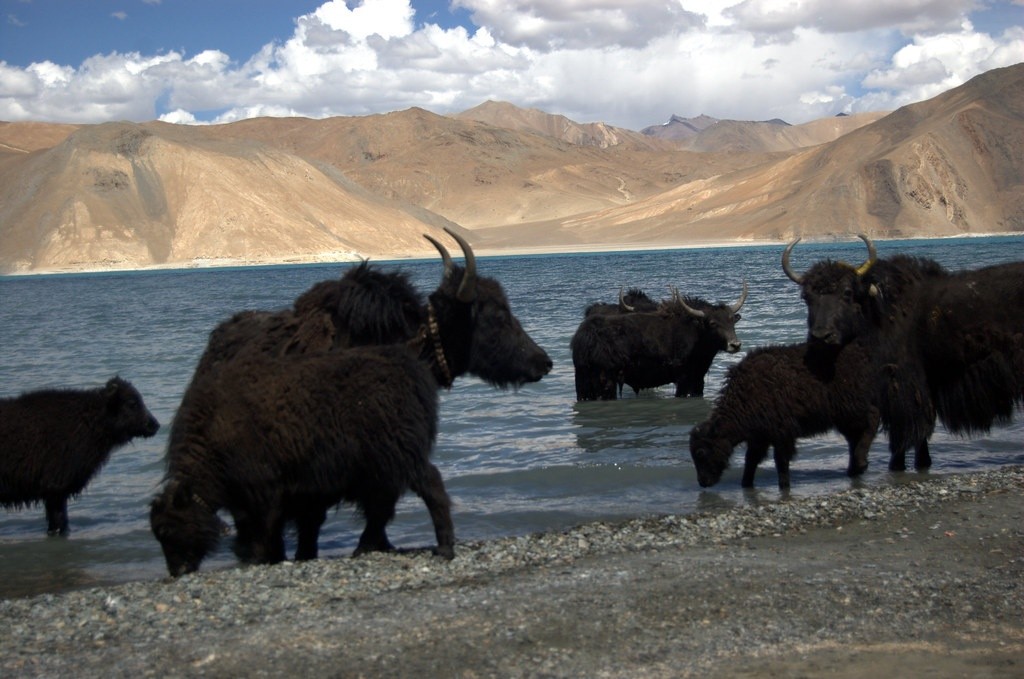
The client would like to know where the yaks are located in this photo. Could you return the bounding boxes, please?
[0,375,161,538]
[145,226,553,579]
[571,233,1023,492]
